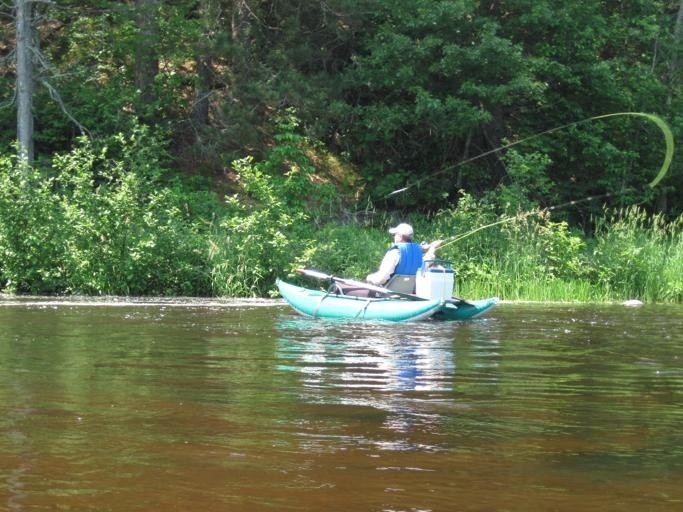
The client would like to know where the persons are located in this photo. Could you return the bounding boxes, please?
[346,223,442,297]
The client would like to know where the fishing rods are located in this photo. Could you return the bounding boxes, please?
[420,183,652,250]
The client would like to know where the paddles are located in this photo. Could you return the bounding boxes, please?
[295,269,458,309]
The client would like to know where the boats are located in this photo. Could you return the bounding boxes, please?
[274,278,497,320]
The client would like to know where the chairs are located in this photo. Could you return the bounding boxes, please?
[376,274,415,298]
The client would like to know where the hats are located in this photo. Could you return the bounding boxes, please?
[388,223,413,240]
